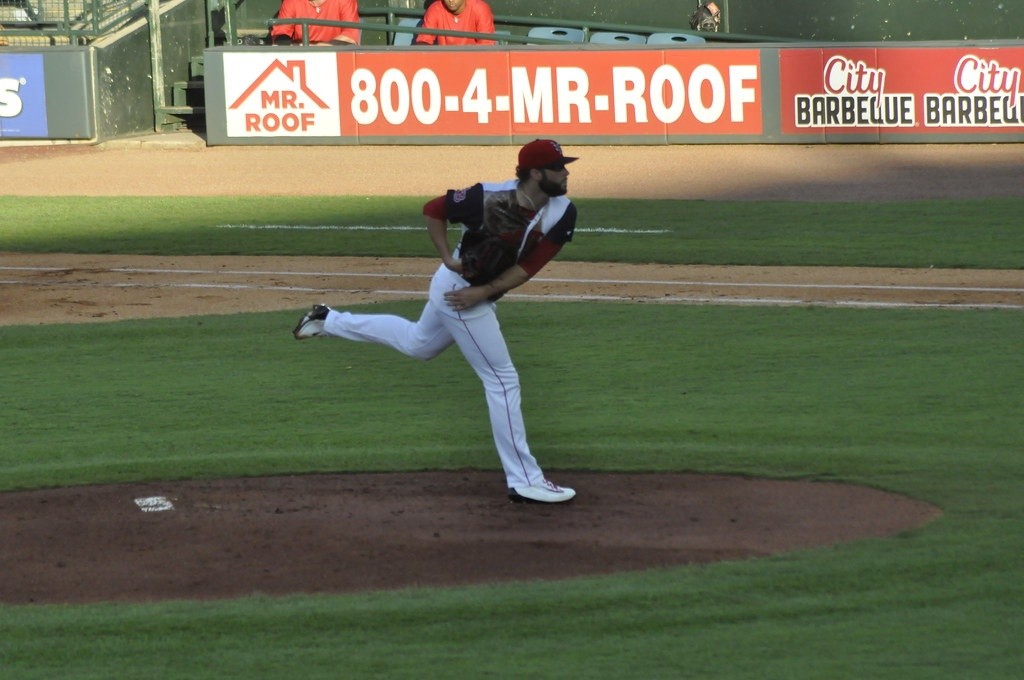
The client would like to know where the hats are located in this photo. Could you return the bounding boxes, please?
[518,139,578,169]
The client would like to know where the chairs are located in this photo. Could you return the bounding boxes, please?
[589,32,647,46]
[524,27,583,46]
[495,30,512,45]
[646,33,706,45]
[393,17,422,48]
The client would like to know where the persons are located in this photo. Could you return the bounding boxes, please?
[294,140,579,500]
[416,0,497,45]
[271,0,361,46]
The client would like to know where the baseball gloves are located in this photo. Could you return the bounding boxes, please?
[462,233,508,286]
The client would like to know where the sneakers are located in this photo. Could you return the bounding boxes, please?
[508,480,576,504]
[293,303,331,340]
